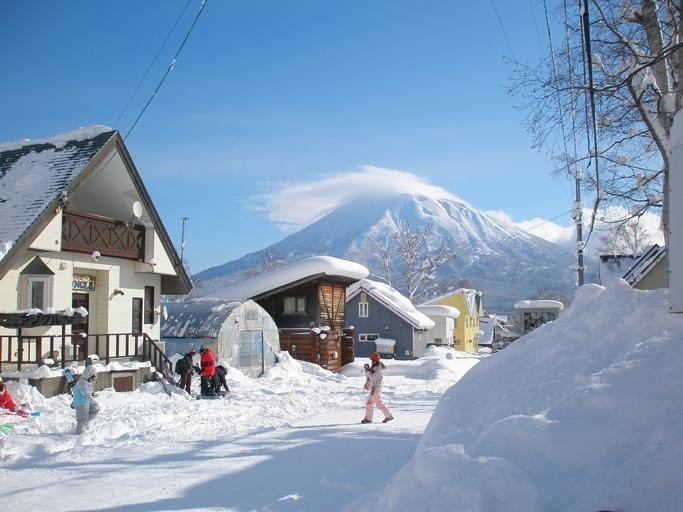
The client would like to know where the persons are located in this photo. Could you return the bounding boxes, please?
[177,348,196,394]
[70,365,98,437]
[196,345,215,396]
[358,351,393,424]
[209,363,229,394]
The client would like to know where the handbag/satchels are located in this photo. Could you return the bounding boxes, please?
[364,378,372,390]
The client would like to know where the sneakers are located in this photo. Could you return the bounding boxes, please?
[381,417,395,423]
[360,418,373,424]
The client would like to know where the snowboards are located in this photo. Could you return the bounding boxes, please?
[63,367,76,397]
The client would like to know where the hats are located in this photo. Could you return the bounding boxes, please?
[189,348,196,353]
[198,345,206,355]
[370,352,381,361]
